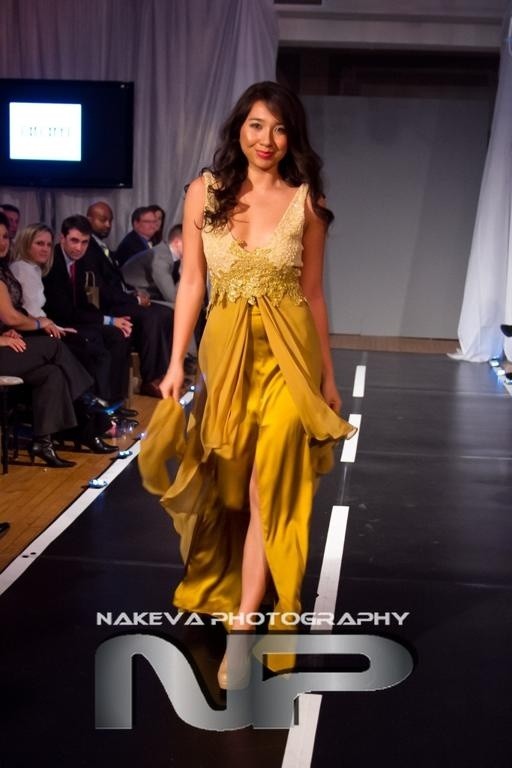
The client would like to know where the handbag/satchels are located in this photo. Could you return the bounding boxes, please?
[83,268,100,310]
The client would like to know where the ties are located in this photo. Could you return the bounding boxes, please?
[102,244,110,258]
[69,263,77,295]
[147,241,153,249]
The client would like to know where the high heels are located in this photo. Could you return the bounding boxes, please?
[28,433,77,468]
[74,430,119,452]
[76,394,125,421]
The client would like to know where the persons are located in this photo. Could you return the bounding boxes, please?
[0,199,209,476]
[140,79,358,692]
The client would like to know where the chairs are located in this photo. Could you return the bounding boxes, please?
[0,376,35,473]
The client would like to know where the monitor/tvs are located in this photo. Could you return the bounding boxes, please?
[0,76,135,189]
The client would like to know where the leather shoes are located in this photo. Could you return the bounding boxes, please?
[140,375,166,399]
[113,406,137,417]
[110,414,139,430]
[217,630,259,690]
